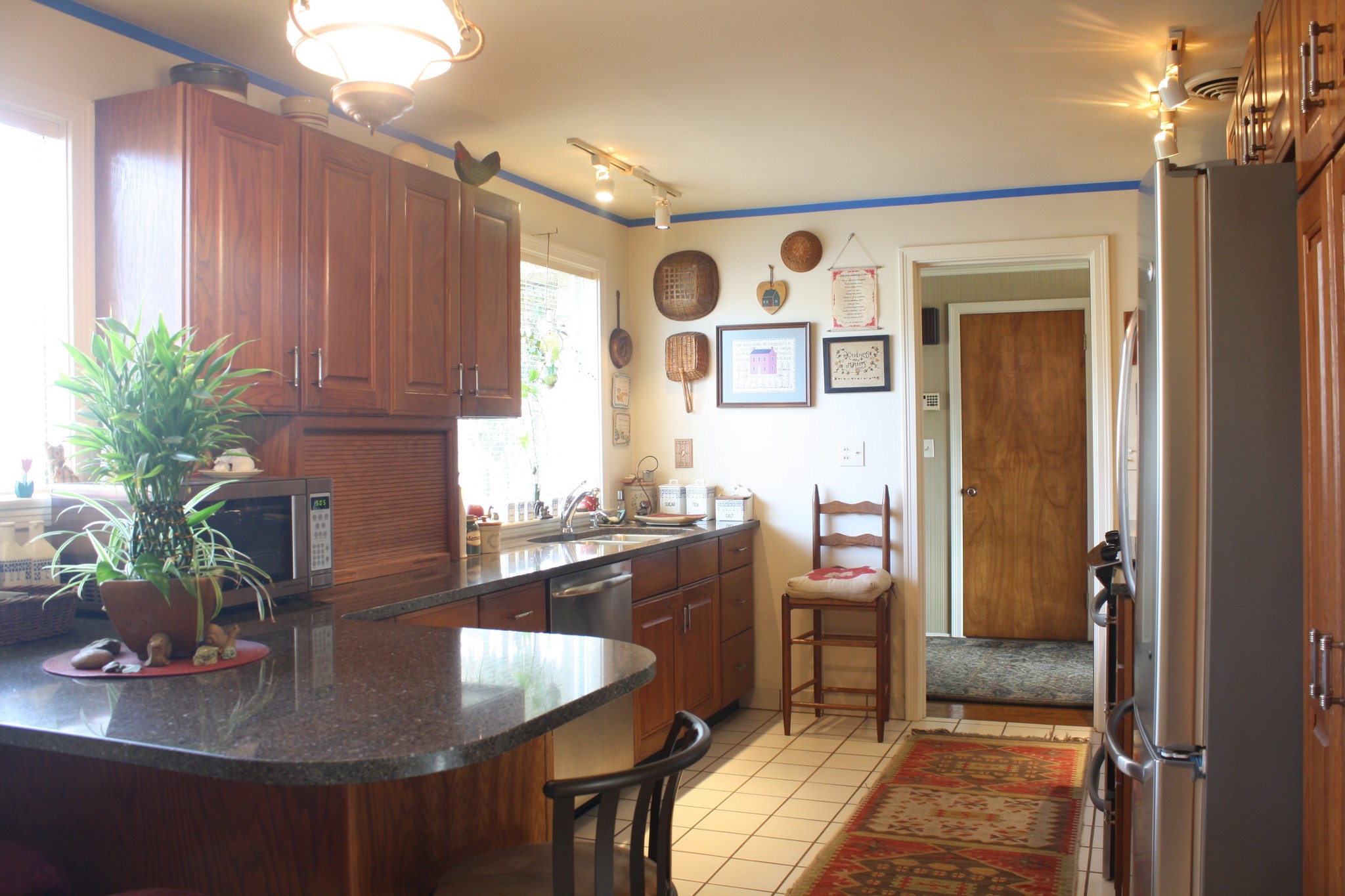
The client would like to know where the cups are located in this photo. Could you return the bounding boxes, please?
[642,471,654,482]
[476,516,502,554]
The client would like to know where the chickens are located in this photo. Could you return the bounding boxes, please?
[454,141,501,187]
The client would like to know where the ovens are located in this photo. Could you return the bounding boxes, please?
[1088,569,1119,880]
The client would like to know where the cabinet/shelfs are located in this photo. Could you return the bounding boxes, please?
[719,529,756,706]
[396,582,549,631]
[97,83,391,416]
[1226,0,1345,896]
[390,157,523,418]
[630,536,720,764]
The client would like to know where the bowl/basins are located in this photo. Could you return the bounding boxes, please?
[279,96,330,132]
[169,63,249,103]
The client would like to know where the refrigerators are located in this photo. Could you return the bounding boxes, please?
[1116,157,1303,896]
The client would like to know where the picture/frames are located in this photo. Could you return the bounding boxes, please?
[823,335,891,393]
[716,322,812,407]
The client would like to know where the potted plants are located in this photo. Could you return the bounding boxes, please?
[24,307,278,655]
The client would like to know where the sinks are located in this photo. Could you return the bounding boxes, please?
[527,527,695,544]
[577,533,673,543]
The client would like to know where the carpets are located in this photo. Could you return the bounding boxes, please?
[782,729,1091,896]
[926,636,1093,708]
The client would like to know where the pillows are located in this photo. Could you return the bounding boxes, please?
[786,566,891,603]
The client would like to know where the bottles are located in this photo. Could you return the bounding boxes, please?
[466,515,481,556]
[0,520,60,589]
[458,487,467,558]
[616,490,625,510]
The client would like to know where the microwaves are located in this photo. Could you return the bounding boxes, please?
[51,476,334,611]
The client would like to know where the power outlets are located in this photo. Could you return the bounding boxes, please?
[838,440,865,466]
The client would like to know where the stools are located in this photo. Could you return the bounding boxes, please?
[782,485,895,741]
[432,711,714,895]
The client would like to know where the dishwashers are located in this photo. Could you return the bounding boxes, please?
[548,559,634,817]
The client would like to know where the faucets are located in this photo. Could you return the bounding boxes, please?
[560,480,600,534]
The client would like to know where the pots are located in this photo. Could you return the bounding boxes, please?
[609,290,633,369]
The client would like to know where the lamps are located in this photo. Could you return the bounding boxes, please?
[592,155,614,201]
[1157,50,1190,109]
[652,186,671,229]
[282,1,483,136]
[1153,111,1178,160]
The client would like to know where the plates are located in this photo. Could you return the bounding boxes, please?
[634,513,708,525]
[197,469,264,478]
